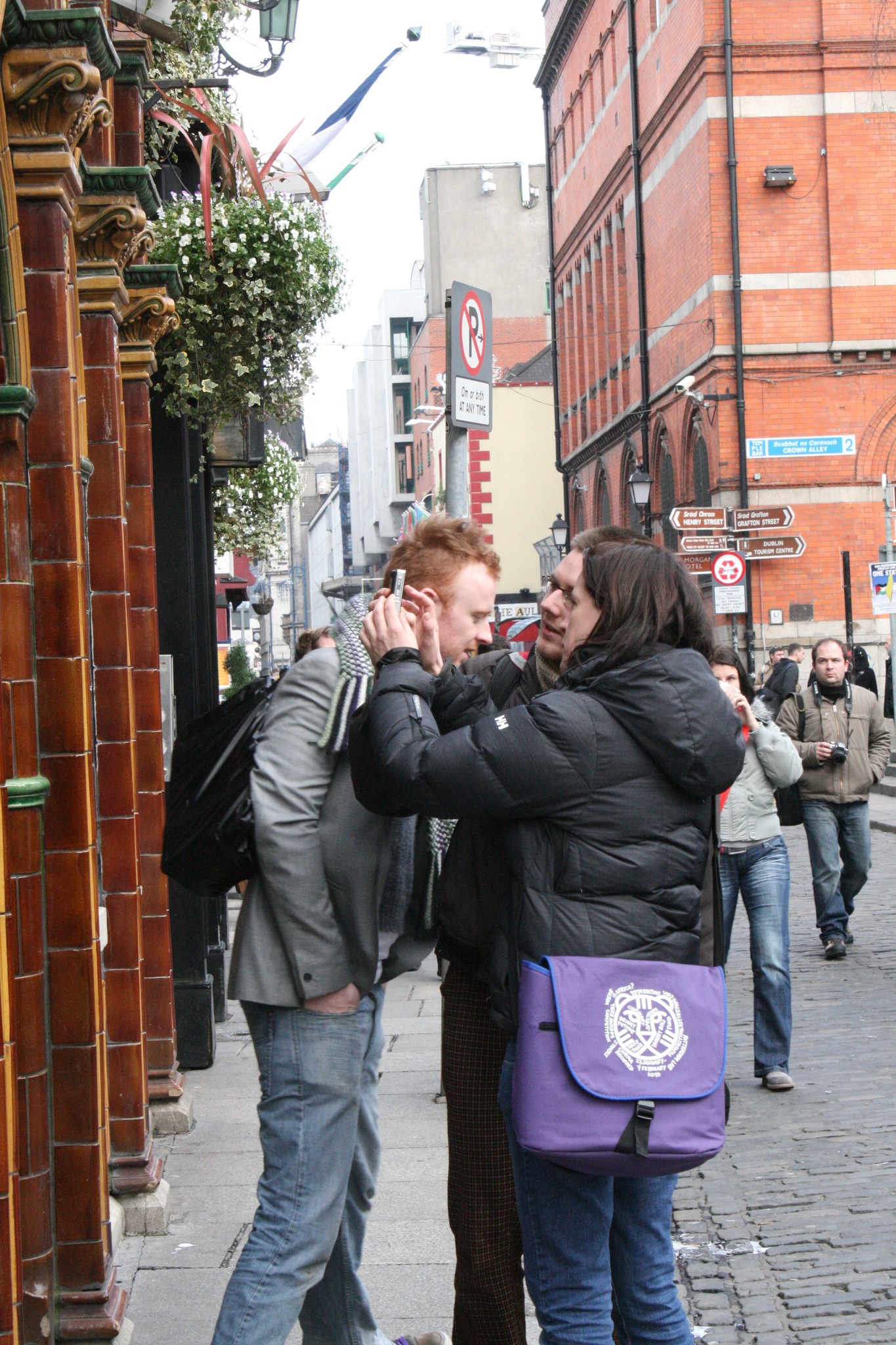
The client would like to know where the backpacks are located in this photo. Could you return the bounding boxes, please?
[162,676,278,897]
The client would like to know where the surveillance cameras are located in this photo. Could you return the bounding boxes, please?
[572,479,579,490]
[675,375,695,393]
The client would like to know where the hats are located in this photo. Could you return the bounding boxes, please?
[854,646,870,670]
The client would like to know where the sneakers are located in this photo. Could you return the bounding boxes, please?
[394,1332,452,1345]
[823,938,847,958]
[845,935,853,944]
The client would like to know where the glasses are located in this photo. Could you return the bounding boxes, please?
[770,646,782,653]
[540,576,576,609]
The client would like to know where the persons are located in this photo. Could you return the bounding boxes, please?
[299,628,895,1092]
[369,521,654,1345]
[214,520,505,1345]
[357,534,753,1345]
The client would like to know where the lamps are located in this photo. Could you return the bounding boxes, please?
[549,514,571,555]
[573,479,587,491]
[482,170,497,192]
[405,418,434,426]
[763,165,796,188]
[625,462,670,519]
[215,0,300,77]
[429,386,446,396]
[414,405,444,414]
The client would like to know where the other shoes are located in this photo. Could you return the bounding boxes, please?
[762,1071,793,1089]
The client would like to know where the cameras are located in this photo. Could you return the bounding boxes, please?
[827,741,848,765]
[387,568,406,614]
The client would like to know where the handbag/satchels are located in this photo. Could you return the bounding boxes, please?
[507,956,731,1174]
[774,783,805,826]
[758,687,784,722]
